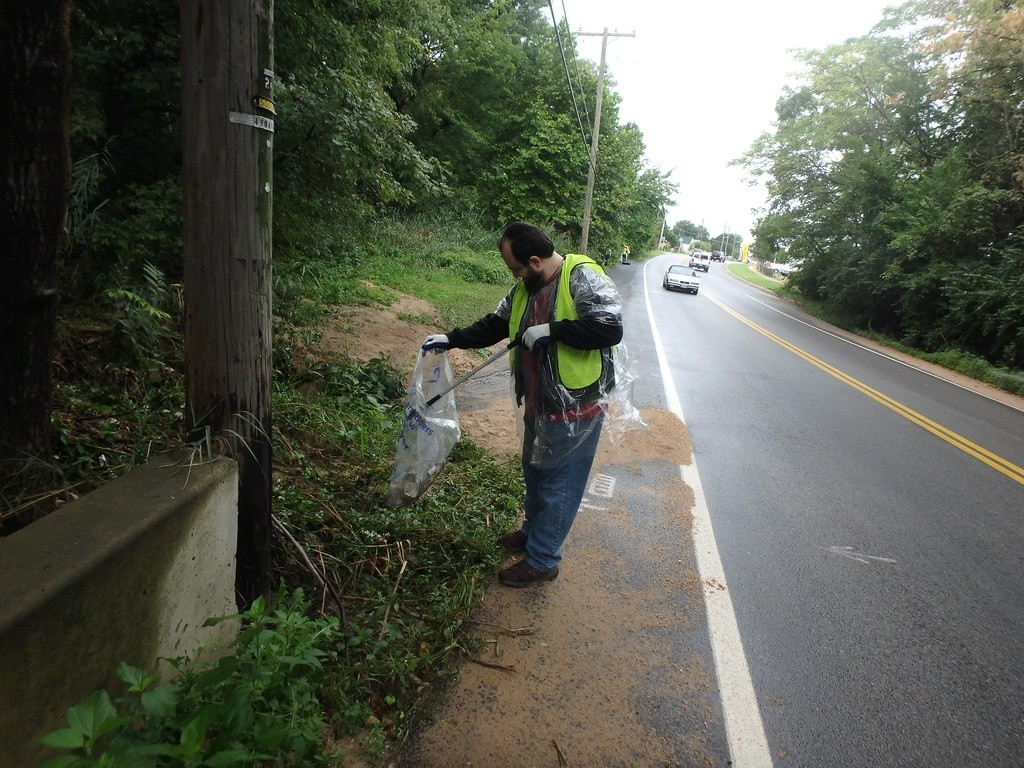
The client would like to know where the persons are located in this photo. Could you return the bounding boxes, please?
[419,221,624,588]
[621,243,632,260]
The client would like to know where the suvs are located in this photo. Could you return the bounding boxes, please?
[711,250,726,263]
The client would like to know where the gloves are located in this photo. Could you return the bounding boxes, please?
[421,334,450,357]
[522,323,551,352]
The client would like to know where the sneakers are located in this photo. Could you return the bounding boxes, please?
[496,530,528,551]
[498,559,559,587]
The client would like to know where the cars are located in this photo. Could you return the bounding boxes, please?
[766,261,791,275]
[663,264,701,295]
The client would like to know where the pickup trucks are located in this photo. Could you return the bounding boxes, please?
[689,252,711,273]
